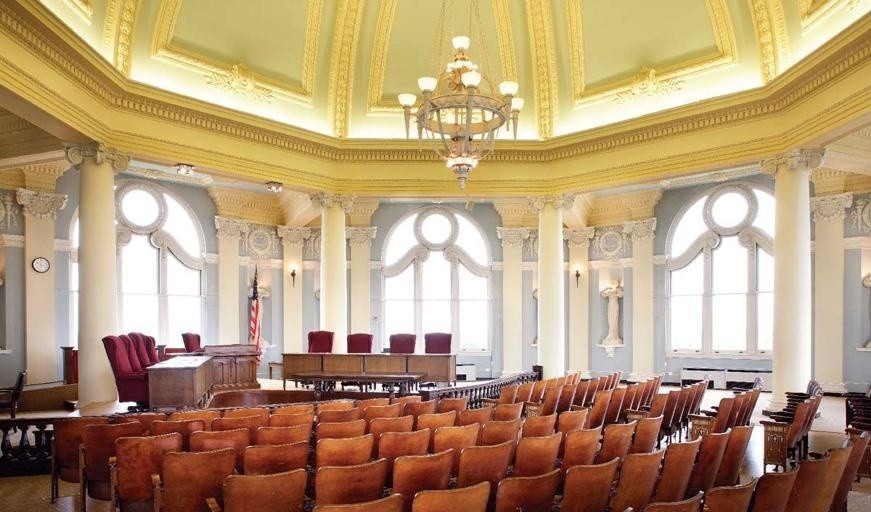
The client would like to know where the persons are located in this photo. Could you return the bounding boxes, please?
[602,278,623,342]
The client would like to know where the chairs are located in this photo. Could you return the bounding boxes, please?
[483,371,824,475]
[844,392,870,483]
[294,334,451,392]
[51,395,469,511]
[182,334,204,356]
[1,370,28,433]
[750,463,801,512]
[828,435,868,512]
[414,401,759,512]
[102,332,160,412]
[788,440,852,512]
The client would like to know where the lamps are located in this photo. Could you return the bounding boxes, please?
[173,163,197,177]
[287,260,300,289]
[574,266,582,288]
[265,181,285,194]
[395,2,522,194]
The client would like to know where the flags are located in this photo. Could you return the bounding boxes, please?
[251,266,263,362]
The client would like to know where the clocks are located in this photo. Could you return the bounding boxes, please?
[32,256,51,274]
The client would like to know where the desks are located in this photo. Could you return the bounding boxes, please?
[204,345,260,391]
[281,353,457,388]
[150,356,212,408]
